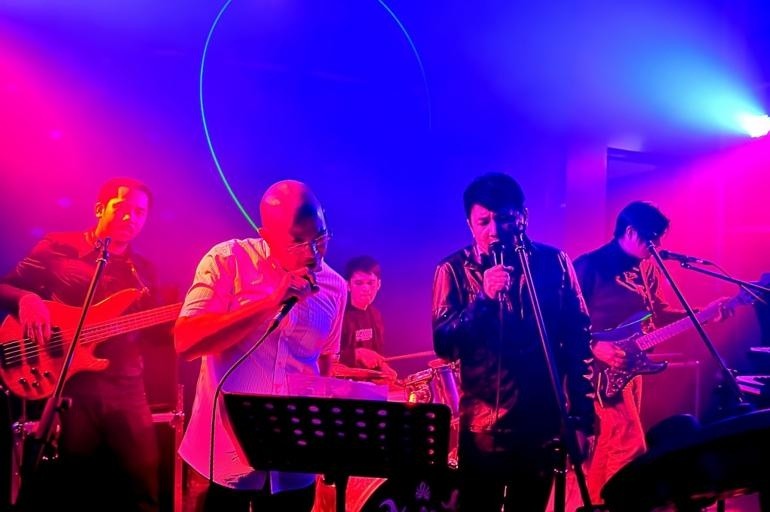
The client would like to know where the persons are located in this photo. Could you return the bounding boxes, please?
[430,170,603,512]
[172,178,346,511]
[572,199,739,506]
[2,175,170,512]
[334,253,398,385]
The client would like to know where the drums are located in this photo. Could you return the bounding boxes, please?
[403,364,462,422]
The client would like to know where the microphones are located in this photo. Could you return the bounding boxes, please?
[488,241,507,302]
[103,238,111,259]
[268,274,313,334]
[660,250,703,264]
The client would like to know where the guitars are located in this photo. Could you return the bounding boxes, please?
[590,282,767,406]
[0,289,185,399]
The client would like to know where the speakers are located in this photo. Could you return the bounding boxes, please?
[15,411,183,512]
[632,358,701,436]
